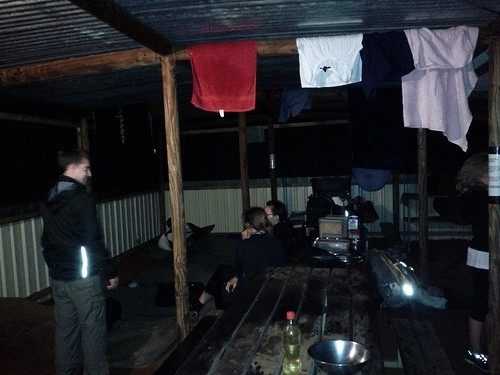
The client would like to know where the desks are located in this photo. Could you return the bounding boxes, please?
[216,266,454,375]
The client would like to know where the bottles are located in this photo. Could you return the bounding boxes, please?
[281,311,302,375]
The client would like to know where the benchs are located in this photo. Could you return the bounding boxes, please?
[154,298,254,375]
[380,222,404,250]
[403,193,450,253]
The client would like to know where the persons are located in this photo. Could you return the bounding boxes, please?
[42,147,120,375]
[189,208,283,318]
[264,200,293,267]
[461,153,489,373]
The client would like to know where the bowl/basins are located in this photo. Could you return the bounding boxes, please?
[306,339,371,375]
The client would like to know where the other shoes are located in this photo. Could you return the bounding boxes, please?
[465,348,491,373]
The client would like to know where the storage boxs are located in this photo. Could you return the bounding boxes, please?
[289,210,307,230]
[318,216,347,238]
